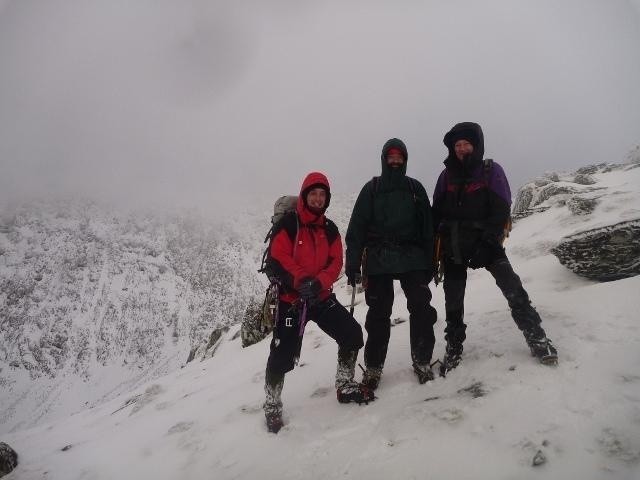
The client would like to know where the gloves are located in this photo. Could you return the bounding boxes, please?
[346,270,362,287]
[297,277,322,299]
[469,236,496,269]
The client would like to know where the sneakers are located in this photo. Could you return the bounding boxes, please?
[411,347,432,376]
[266,414,284,432]
[523,325,559,363]
[362,366,384,387]
[337,381,374,401]
[443,343,463,369]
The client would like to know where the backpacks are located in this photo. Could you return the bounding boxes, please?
[263,195,299,284]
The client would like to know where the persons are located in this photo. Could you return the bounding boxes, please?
[431,122,561,376]
[344,137,438,388]
[258,170,364,435]
